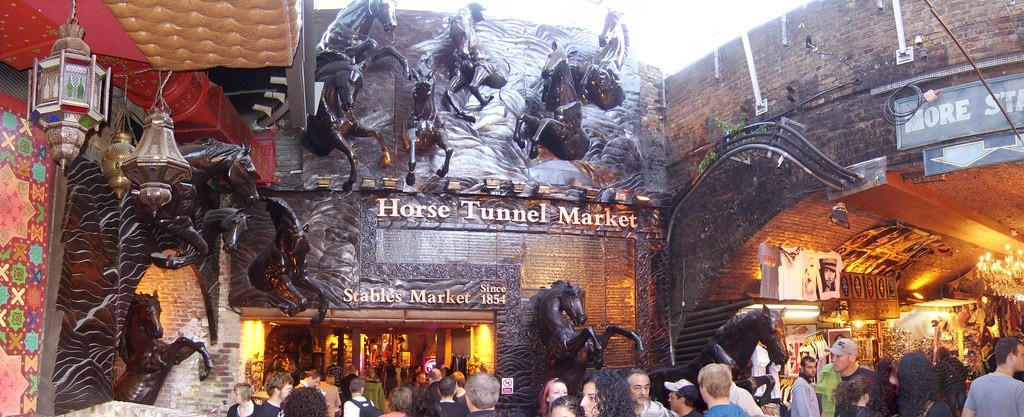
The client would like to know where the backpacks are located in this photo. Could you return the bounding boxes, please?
[348,396,382,417]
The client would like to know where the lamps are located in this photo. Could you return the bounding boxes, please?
[102,76,137,198]
[26,0,112,171]
[121,70,193,217]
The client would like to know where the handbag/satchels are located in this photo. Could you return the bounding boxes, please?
[955,372,966,384]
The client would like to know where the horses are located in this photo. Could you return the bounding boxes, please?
[301,47,393,192]
[149,138,261,271]
[534,280,645,396]
[402,68,453,186]
[571,8,629,111]
[114,290,215,405]
[647,302,789,408]
[315,0,410,80]
[445,17,507,123]
[513,39,590,160]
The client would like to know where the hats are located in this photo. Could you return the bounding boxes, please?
[824,338,857,356]
[450,372,465,380]
[664,379,698,401]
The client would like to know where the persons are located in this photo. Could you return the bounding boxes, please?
[226,358,525,417]
[537,363,766,417]
[961,337,1024,417]
[791,356,821,417]
[816,337,1000,417]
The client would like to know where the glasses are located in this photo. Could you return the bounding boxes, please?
[1016,338,1023,349]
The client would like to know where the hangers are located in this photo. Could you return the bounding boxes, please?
[801,330,824,349]
[780,378,796,389]
[760,237,839,258]
